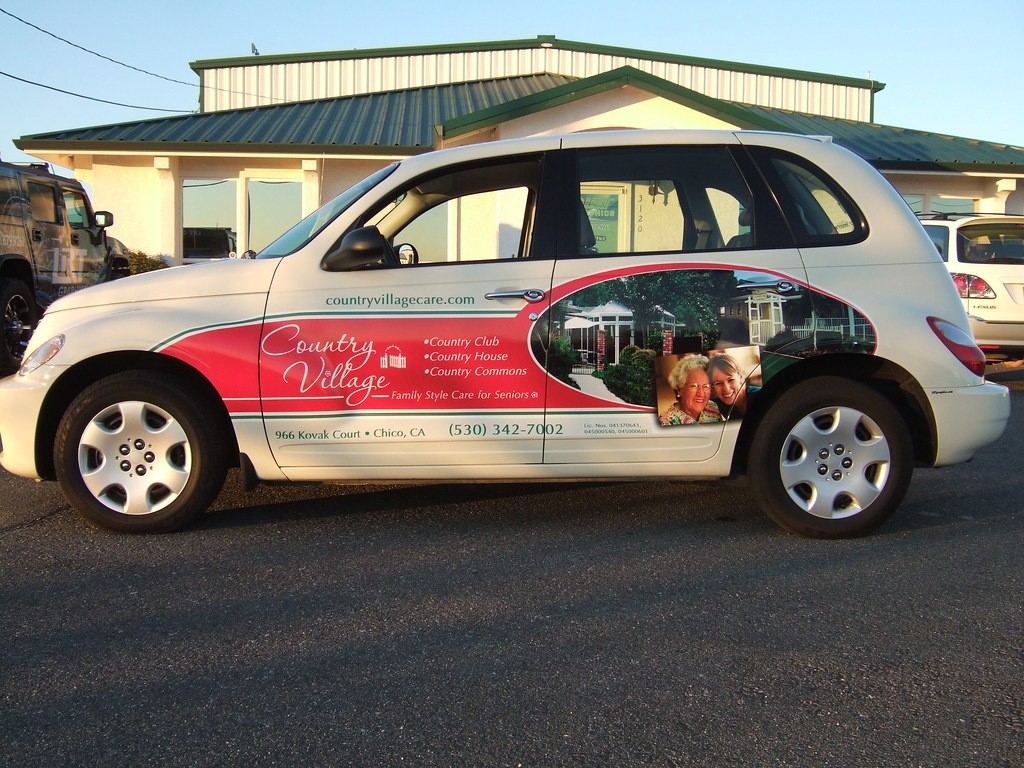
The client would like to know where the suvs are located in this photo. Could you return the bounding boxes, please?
[0,159,131,378]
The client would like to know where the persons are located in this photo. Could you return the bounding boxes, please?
[658,352,762,425]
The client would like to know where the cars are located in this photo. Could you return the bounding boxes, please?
[910,208,1024,357]
[0,131,1014,535]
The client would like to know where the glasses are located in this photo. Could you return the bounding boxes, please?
[684,383,714,393]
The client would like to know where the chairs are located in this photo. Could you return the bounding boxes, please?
[579,203,597,257]
[725,210,755,248]
[967,244,999,259]
